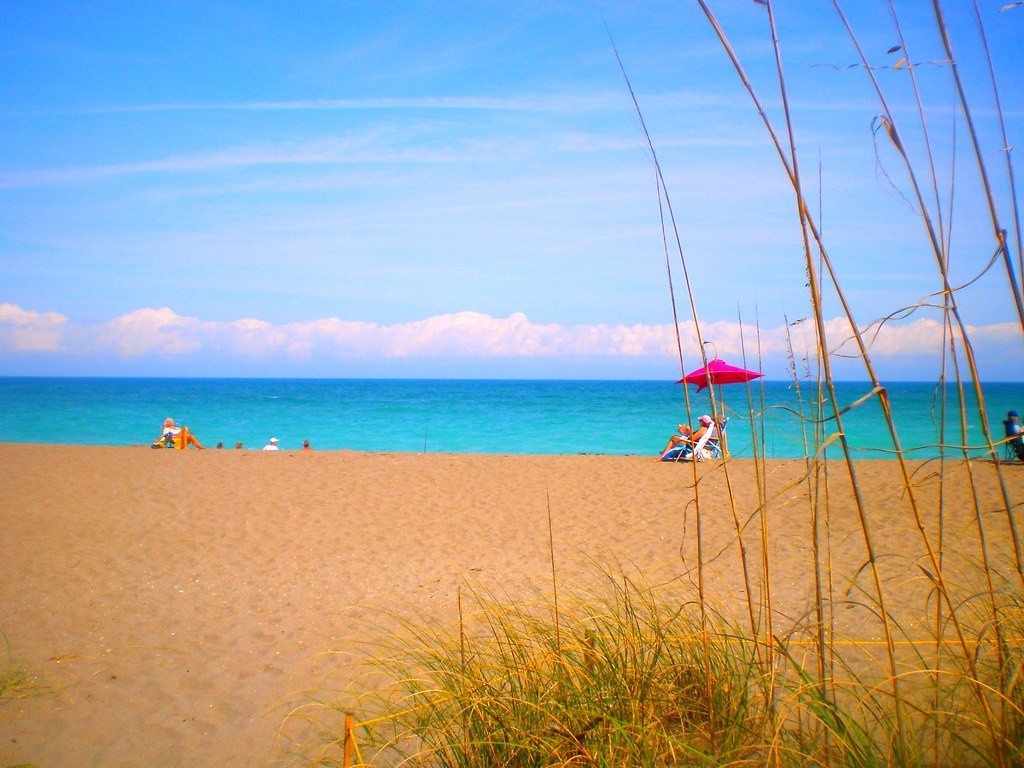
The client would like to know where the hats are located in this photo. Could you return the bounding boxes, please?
[697,414,711,424]
[270,437,279,443]
[717,414,725,425]
[1008,410,1020,418]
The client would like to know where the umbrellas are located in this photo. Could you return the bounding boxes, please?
[675,359,767,458]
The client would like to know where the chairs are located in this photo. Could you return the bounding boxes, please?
[659,417,732,463]
[159,423,193,450]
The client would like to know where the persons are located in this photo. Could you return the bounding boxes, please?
[1007,410,1024,450]
[217,442,223,449]
[162,418,206,450]
[659,415,725,455]
[302,440,313,451]
[262,437,279,450]
[236,441,242,449]
[658,415,712,461]
